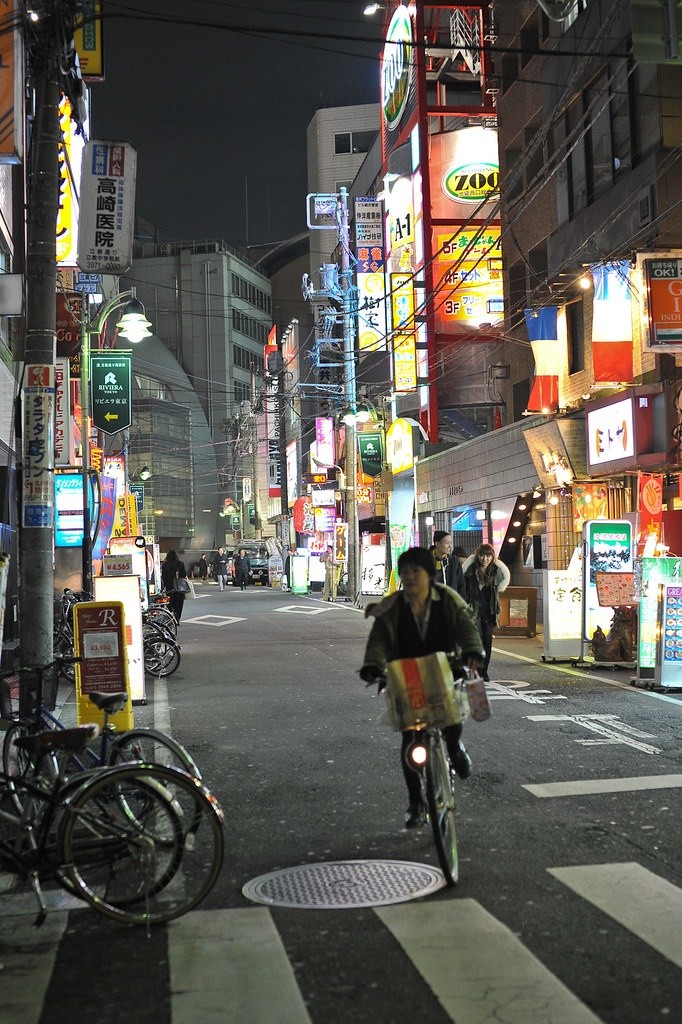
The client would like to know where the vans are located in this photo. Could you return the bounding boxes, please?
[192,543,269,587]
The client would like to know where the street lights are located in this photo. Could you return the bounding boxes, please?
[81,288,153,600]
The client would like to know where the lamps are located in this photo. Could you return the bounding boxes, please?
[364,3,386,16]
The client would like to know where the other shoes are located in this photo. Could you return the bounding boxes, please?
[479,668,489,682]
[449,743,472,780]
[405,800,427,830]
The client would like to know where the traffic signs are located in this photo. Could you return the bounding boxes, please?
[90,354,132,436]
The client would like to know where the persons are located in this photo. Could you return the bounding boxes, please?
[429,530,466,600]
[285,549,294,592]
[463,544,511,682]
[360,546,484,827]
[162,551,186,625]
[235,549,251,591]
[200,554,209,579]
[453,546,467,565]
[214,547,229,592]
[320,546,340,602]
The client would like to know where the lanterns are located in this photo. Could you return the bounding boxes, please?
[294,495,313,535]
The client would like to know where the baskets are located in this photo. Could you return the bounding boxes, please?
[383,683,467,731]
[54,602,63,624]
[1,664,59,720]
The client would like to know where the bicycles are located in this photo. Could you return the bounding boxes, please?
[354,661,469,886]
[52,588,183,683]
[0,657,227,937]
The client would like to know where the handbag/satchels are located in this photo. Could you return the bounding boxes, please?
[458,664,491,721]
[174,576,191,594]
[384,648,463,735]
